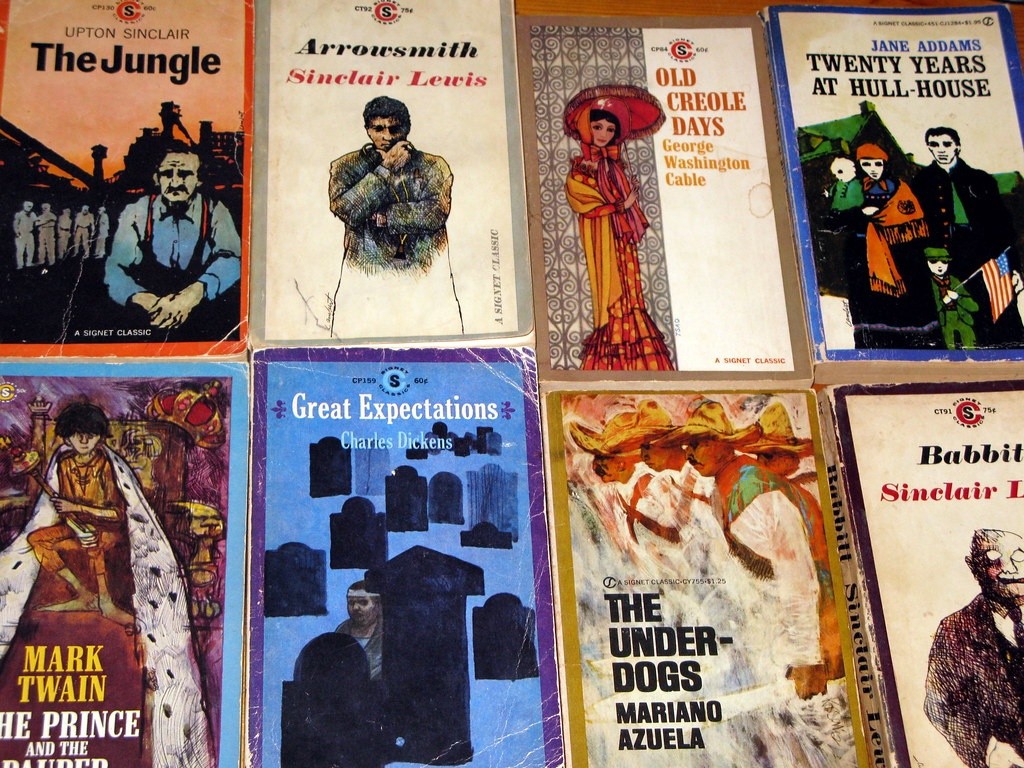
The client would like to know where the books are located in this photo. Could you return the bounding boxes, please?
[0,0,1024,768]
